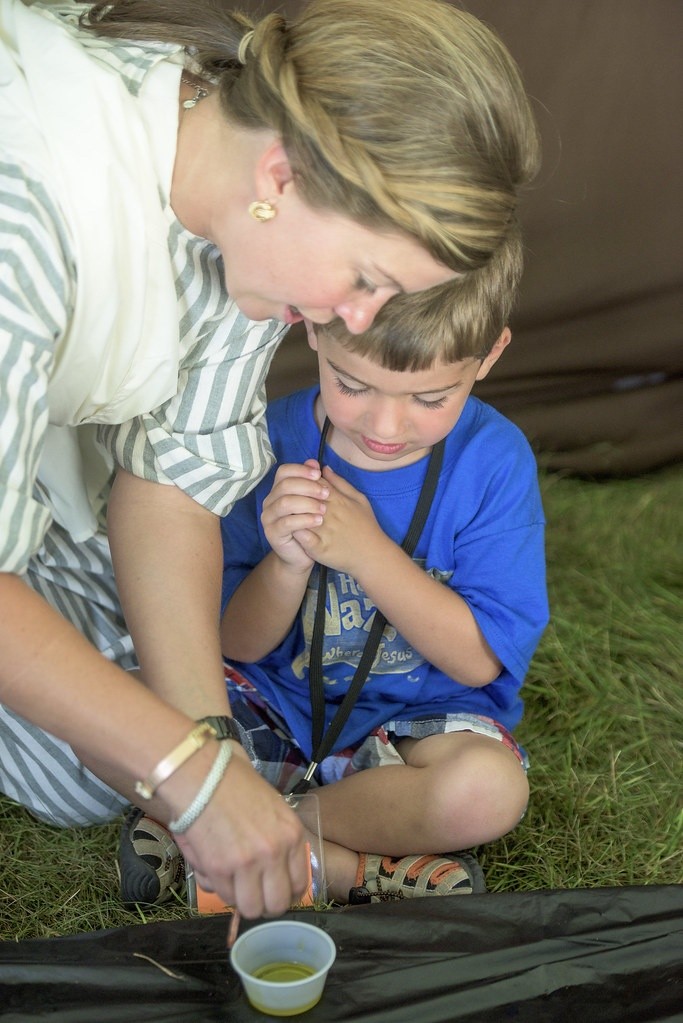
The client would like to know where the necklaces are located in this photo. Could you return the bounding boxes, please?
[179,77,210,137]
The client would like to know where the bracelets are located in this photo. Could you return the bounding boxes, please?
[134,722,216,800]
[167,739,232,833]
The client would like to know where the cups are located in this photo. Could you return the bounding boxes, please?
[227,921,336,1017]
[184,796,326,919]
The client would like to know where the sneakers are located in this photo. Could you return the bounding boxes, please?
[118,807,180,908]
[350,849,488,901]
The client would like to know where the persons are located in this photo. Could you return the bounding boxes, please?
[0,0,542,920]
[110,215,548,914]
